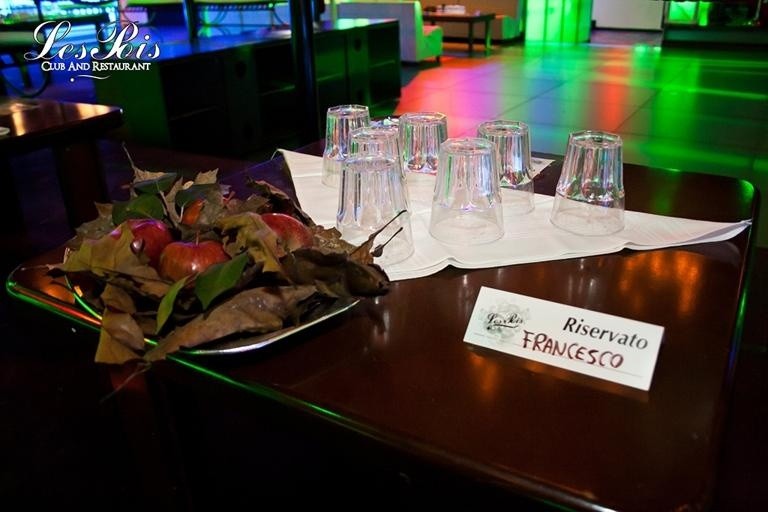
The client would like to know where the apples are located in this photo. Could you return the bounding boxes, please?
[183,190,236,225]
[159,224,231,282]
[256,213,314,256]
[110,217,172,265]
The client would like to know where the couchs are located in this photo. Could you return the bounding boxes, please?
[444,0,524,45]
[338,0,442,64]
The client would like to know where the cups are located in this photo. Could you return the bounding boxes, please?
[397,110,450,206]
[345,125,413,212]
[435,3,466,14]
[334,154,416,268]
[550,129,625,239]
[475,120,534,218]
[427,136,506,248]
[321,103,371,191]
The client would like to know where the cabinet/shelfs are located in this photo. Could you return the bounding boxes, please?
[91,17,403,148]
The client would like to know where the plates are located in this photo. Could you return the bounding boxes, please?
[63,245,362,359]
